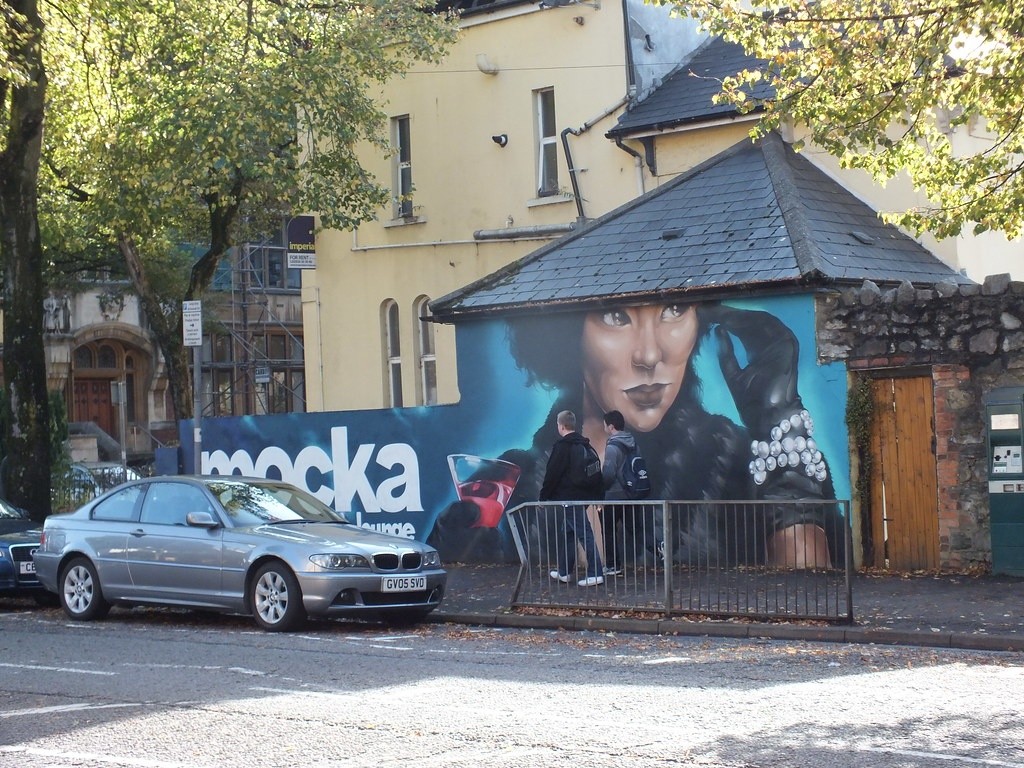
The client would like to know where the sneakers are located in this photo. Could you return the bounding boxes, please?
[550,571,571,582]
[602,567,622,575]
[658,541,665,560]
[578,575,604,587]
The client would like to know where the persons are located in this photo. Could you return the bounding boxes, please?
[538,410,604,586]
[597,410,664,576]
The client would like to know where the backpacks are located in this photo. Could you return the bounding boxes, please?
[558,439,602,488]
[606,440,651,500]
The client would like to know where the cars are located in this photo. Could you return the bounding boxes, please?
[0,497,62,608]
[33,475,448,632]
[49,461,145,514]
